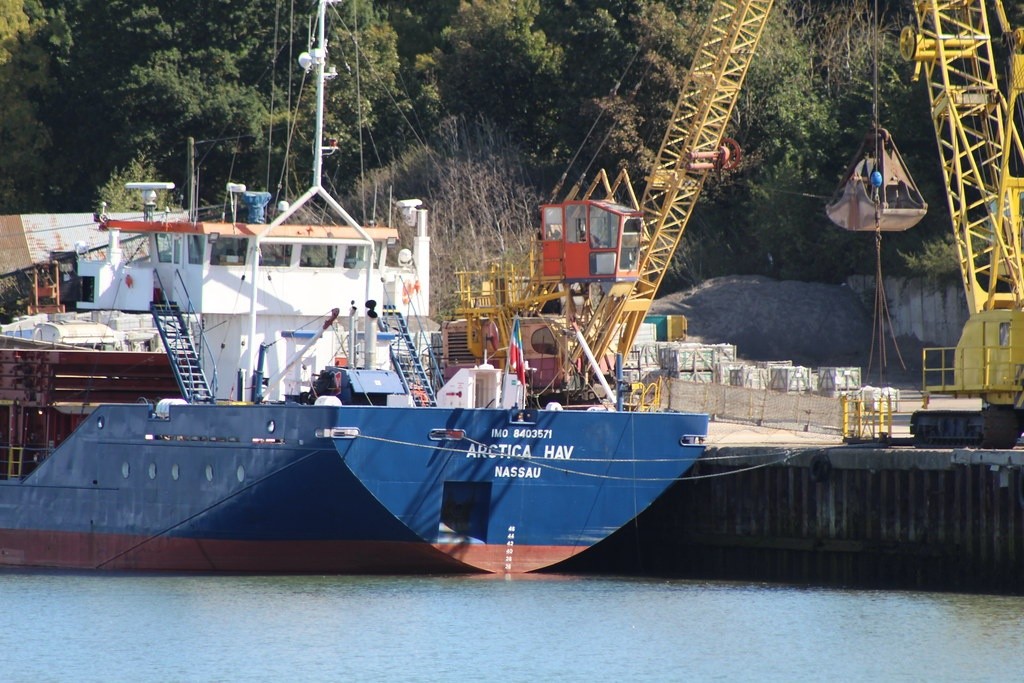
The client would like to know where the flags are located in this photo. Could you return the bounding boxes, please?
[509,319,526,387]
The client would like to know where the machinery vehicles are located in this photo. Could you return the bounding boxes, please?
[424,0,778,409]
[893,0,1024,449]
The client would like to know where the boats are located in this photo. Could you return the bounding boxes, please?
[1,0,712,577]
